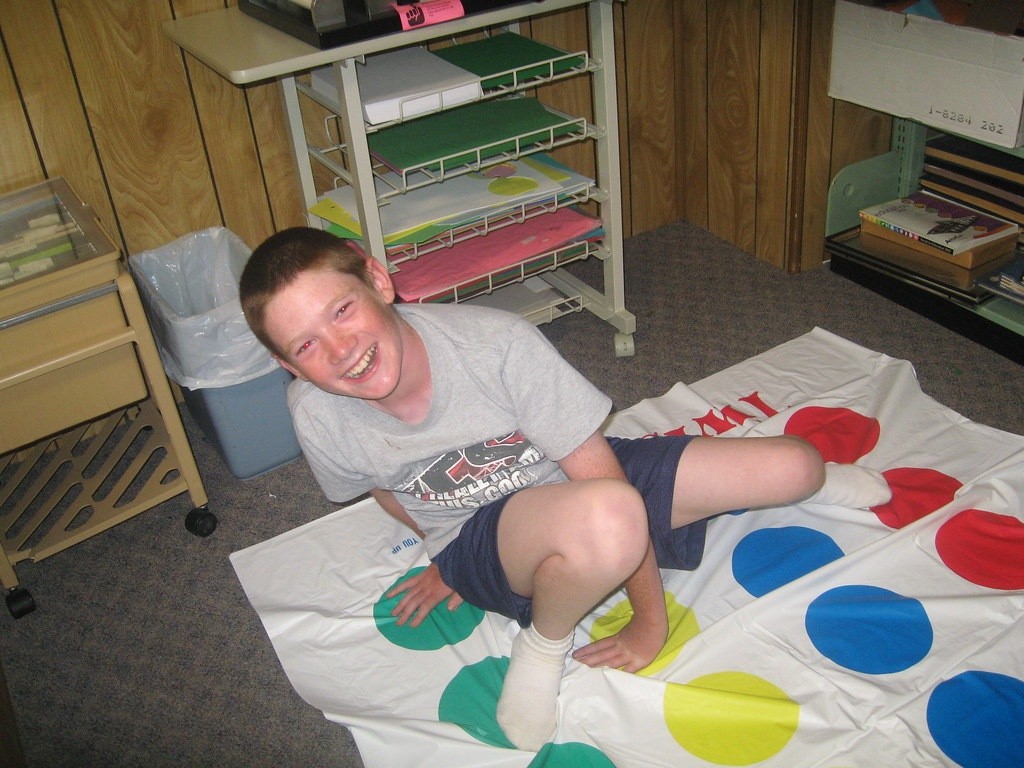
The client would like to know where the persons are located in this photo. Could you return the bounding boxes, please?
[240,228,893,752]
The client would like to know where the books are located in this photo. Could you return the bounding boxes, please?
[857,189,1024,310]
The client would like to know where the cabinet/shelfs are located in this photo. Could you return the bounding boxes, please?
[0,173,217,617]
[160,0,640,359]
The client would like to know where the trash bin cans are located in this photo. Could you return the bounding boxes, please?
[128,226,305,483]
